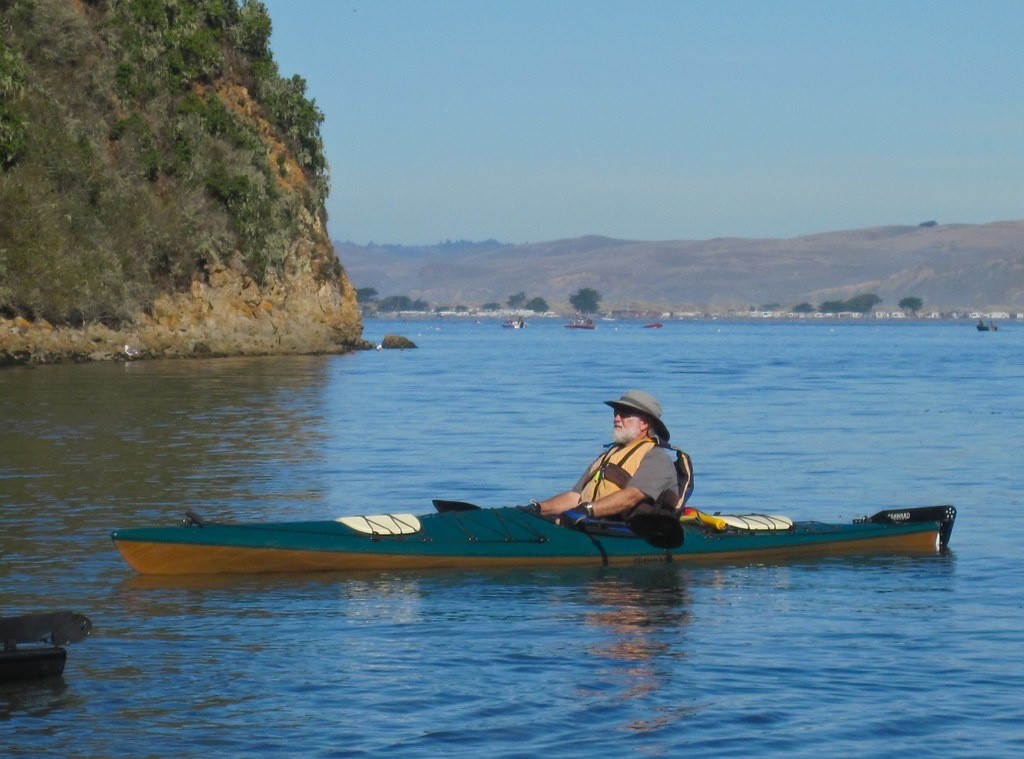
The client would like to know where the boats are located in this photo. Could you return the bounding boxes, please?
[976,325,999,331]
[501,322,529,327]
[109,501,958,579]
[564,324,599,330]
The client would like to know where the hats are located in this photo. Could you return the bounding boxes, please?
[603,390,670,443]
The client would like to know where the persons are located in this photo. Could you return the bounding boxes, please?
[576,316,594,327]
[509,318,526,328]
[527,388,695,536]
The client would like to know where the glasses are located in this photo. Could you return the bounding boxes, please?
[612,408,640,419]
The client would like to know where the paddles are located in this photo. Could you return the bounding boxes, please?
[432,498,684,550]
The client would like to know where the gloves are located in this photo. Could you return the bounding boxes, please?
[522,501,542,516]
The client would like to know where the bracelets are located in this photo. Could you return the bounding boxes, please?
[584,503,595,514]
